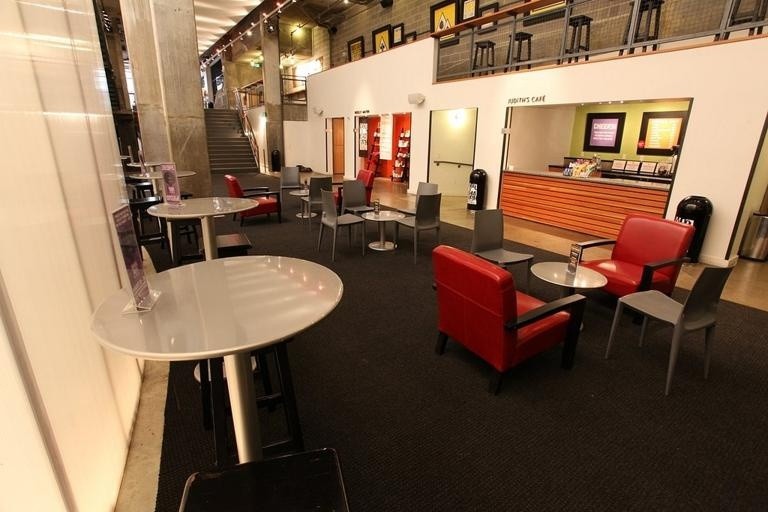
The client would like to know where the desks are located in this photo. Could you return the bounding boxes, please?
[90,161,346,463]
[530,261,609,300]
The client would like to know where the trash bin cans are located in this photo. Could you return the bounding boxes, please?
[674,196,713,264]
[739,213,768,262]
[271,150,281,172]
[467,169,485,210]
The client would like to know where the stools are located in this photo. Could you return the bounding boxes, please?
[618,0,665,56]
[556,14,593,65]
[471,40,496,77]
[714,0,768,41]
[177,447,351,512]
[503,32,533,73]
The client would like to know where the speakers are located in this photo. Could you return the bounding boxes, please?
[380,0,393,8]
[331,27,337,33]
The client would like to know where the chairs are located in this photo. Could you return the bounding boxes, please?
[318,170,442,265]
[574,213,697,325]
[471,210,534,295]
[431,245,587,396]
[605,267,733,396]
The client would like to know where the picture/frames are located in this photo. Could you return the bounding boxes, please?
[347,0,574,63]
